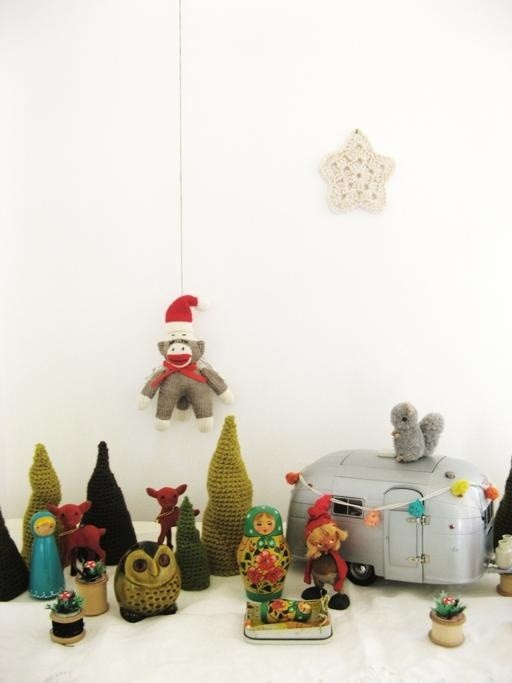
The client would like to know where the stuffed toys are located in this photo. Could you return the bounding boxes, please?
[390,401,445,462]
[137,295,233,432]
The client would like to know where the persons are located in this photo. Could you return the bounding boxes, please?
[301,494,350,610]
[28,512,66,599]
